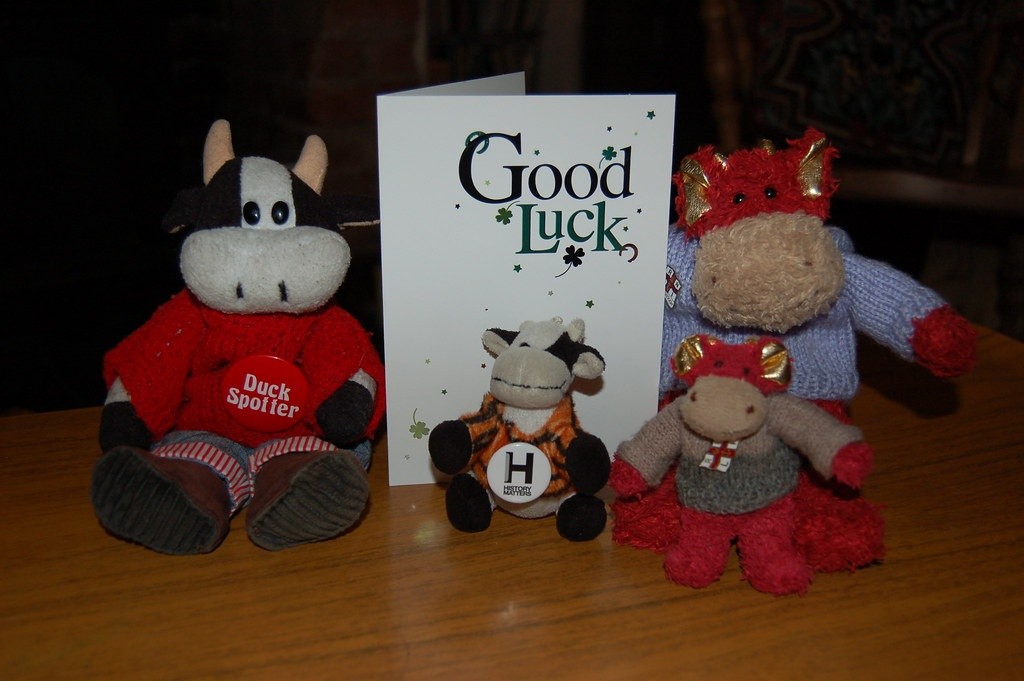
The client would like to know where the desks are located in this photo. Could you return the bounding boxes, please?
[0,321,1024,681]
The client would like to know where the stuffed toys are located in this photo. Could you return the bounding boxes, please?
[607,128,979,576]
[91,119,385,555]
[606,333,872,598]
[429,315,612,542]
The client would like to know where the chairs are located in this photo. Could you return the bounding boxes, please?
[702,0,1024,339]
